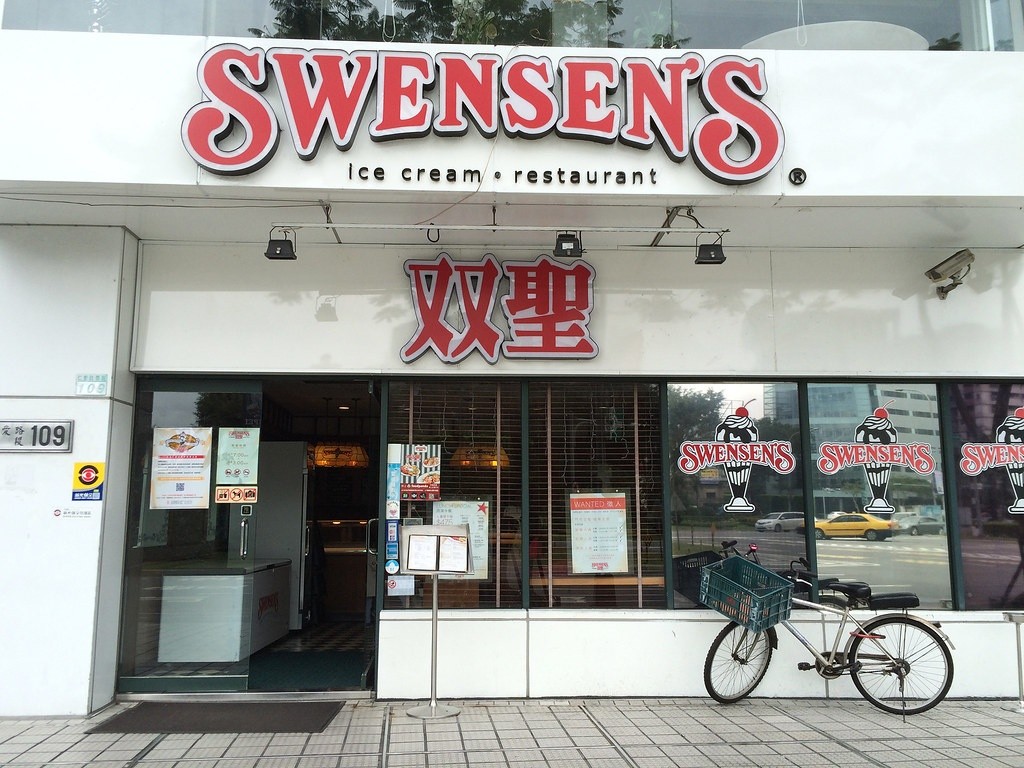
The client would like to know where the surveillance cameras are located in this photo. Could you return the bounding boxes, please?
[924,248,976,282]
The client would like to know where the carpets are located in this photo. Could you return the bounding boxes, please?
[85,699,347,733]
[250,651,373,689]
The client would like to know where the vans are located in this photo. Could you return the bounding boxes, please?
[893,512,917,522]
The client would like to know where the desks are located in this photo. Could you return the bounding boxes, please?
[530,576,666,585]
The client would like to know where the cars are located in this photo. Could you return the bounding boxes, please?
[796,514,902,542]
[827,511,847,519]
[755,511,818,533]
[899,516,946,536]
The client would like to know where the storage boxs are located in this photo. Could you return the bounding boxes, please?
[673,550,724,602]
[699,555,795,632]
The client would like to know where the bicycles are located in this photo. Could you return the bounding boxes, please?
[699,556,955,724]
[671,539,855,611]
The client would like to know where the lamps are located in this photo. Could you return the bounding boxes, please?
[553,230,587,257]
[315,415,370,468]
[448,443,509,469]
[264,226,298,260]
[694,232,727,265]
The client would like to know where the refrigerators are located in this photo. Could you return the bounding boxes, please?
[157,558,293,664]
[227,440,318,631]
